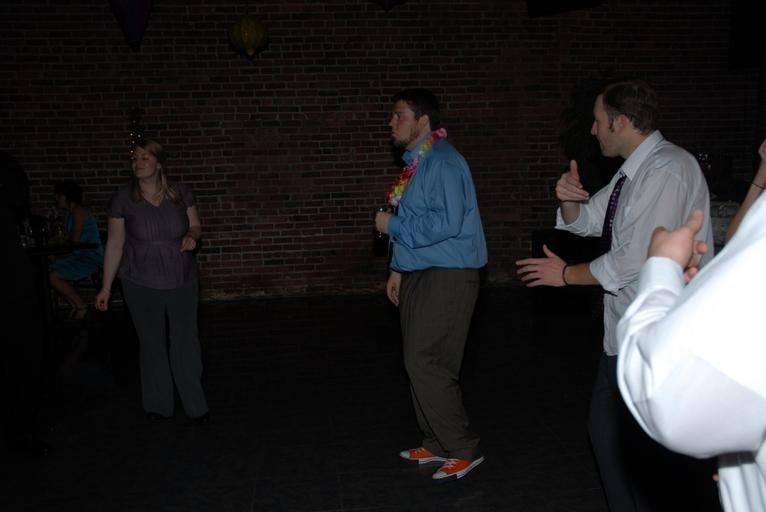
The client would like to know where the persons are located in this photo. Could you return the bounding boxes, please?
[615,192,766,512]
[514,79,715,512]
[94,138,212,429]
[375,86,488,484]
[0,152,105,323]
[726,140,766,244]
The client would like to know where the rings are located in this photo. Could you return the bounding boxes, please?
[104,303,109,307]
[190,246,194,251]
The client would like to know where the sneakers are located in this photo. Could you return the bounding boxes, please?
[399,447,486,483]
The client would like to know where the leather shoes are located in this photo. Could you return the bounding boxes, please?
[68,305,90,320]
[146,412,210,423]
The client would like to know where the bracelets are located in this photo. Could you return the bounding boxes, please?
[561,263,571,288]
[101,287,112,296]
[752,183,765,191]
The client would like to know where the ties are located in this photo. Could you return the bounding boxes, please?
[602,174,627,250]
